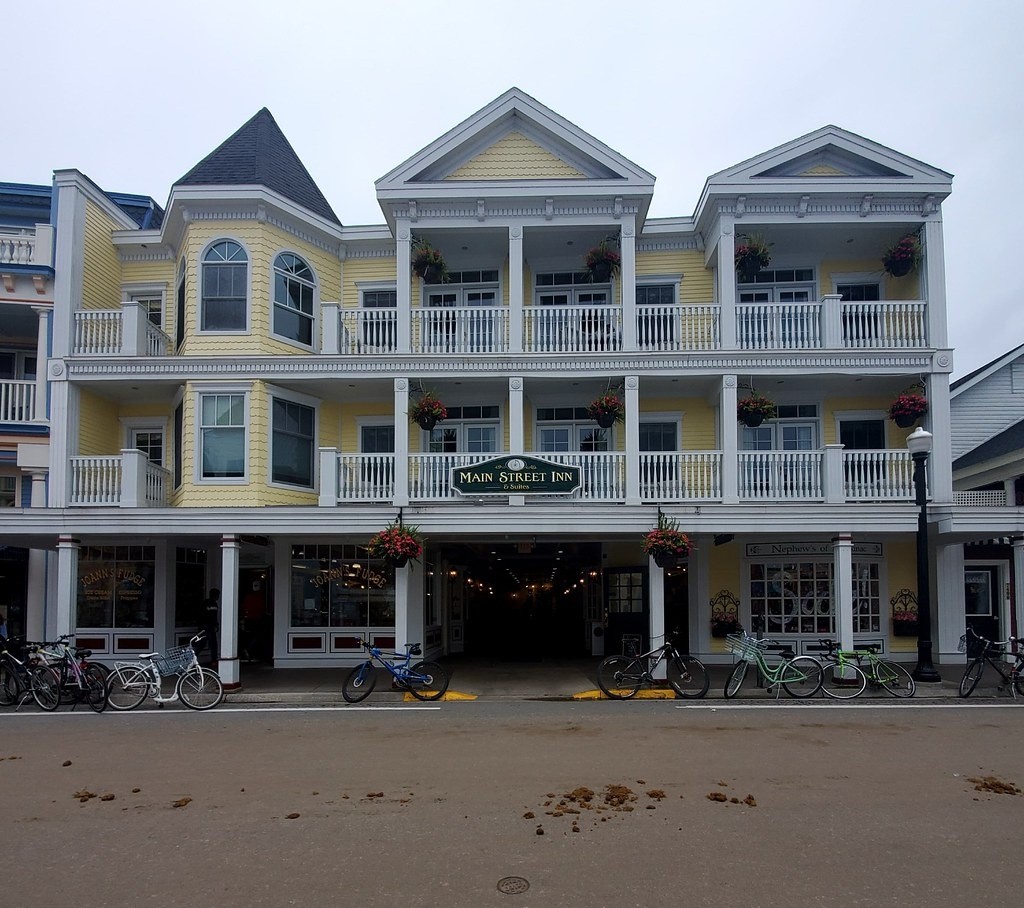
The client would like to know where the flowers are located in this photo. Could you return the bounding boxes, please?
[893,611,918,620]
[735,231,923,276]
[411,235,620,285]
[712,612,741,625]
[403,386,627,423]
[737,389,928,423]
[368,521,425,570]
[640,518,697,556]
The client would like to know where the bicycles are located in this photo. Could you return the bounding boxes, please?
[341,636,449,704]
[957,627,1024,698]
[597,625,710,700]
[723,628,825,700]
[102,630,224,711]
[0,634,114,712]
[816,639,917,699]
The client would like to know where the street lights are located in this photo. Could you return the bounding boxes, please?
[906,426,941,682]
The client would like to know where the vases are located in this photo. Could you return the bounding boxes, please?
[387,555,408,568]
[597,415,614,428]
[894,620,921,637]
[892,260,908,277]
[593,264,610,283]
[419,416,435,430]
[743,261,761,276]
[896,414,917,428]
[745,415,763,427]
[423,265,443,284]
[710,623,742,639]
[654,553,679,568]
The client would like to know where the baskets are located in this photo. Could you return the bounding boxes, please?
[958,634,987,658]
[724,633,769,662]
[154,644,195,677]
